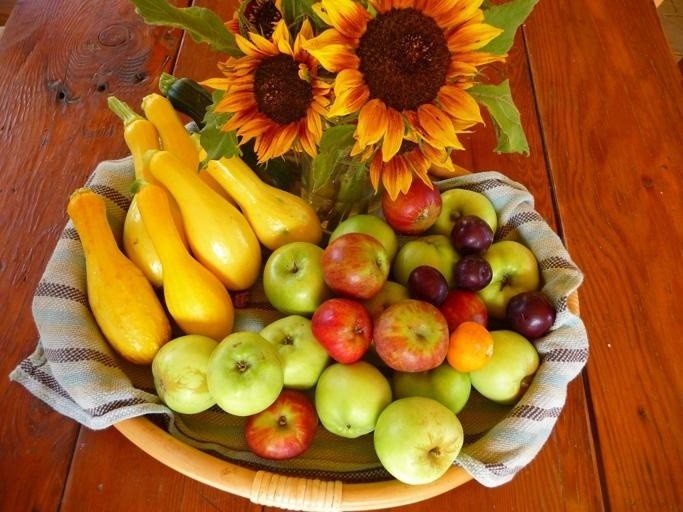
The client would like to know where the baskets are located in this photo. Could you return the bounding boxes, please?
[32,110,581,508]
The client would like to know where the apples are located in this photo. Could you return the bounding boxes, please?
[151,176,557,486]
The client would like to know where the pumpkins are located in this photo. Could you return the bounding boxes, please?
[66,72,322,365]
